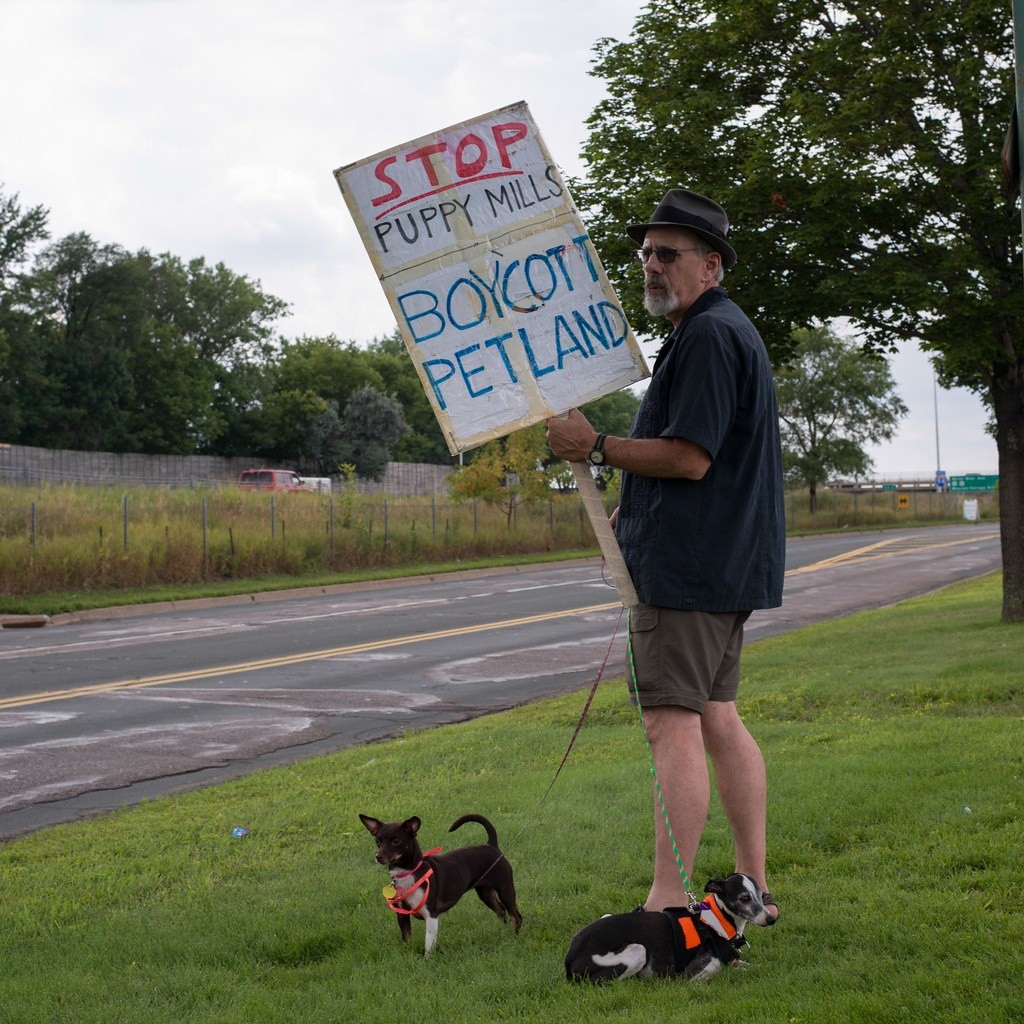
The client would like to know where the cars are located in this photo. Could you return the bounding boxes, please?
[236,468,316,493]
[548,464,614,492]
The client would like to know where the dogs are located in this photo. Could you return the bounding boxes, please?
[565,873,776,988]
[358,814,523,958]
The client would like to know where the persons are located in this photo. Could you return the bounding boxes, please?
[541,183,788,935]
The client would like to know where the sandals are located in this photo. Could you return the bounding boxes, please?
[751,892,781,927]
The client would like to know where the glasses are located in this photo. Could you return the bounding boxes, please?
[635,248,704,264]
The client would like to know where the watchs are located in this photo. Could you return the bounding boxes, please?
[589,433,607,468]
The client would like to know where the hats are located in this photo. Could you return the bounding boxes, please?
[625,188,737,270]
